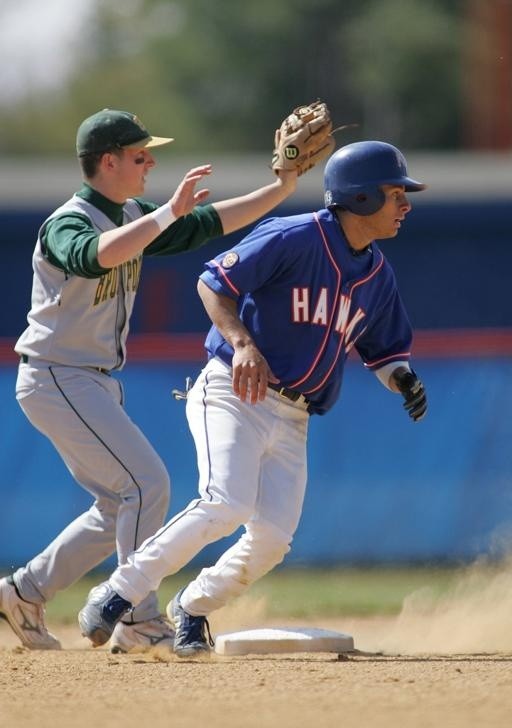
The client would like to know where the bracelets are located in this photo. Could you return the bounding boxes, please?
[151,202,177,233]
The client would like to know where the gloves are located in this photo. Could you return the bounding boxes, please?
[396,368,429,422]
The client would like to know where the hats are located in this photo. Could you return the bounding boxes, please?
[75,109,174,153]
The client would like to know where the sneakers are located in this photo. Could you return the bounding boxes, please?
[1,575,62,650]
[79,580,213,659]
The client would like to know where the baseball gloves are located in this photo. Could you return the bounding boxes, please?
[270,101,336,178]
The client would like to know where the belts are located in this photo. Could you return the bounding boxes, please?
[269,383,314,415]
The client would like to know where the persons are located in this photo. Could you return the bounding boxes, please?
[76,139,433,658]
[0,93,337,655]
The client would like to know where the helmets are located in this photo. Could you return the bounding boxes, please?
[324,141,427,217]
